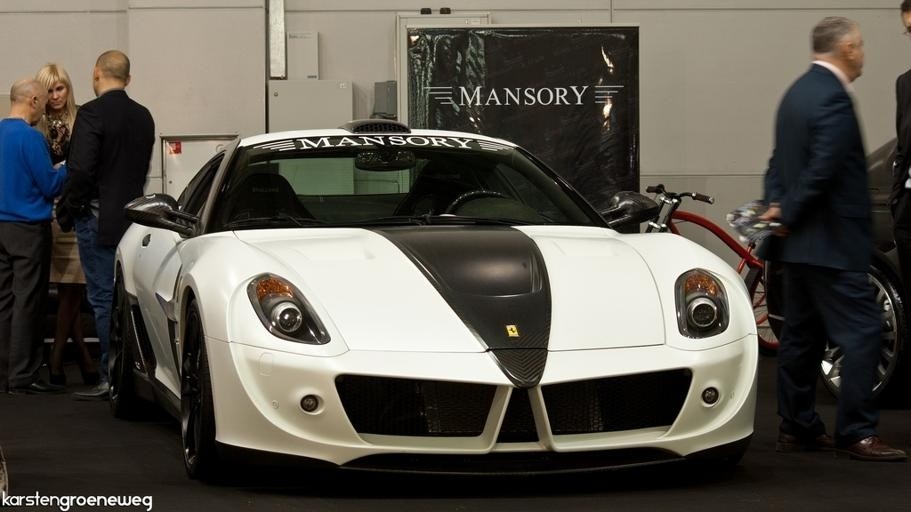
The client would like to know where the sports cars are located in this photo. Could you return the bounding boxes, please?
[108,119,759,477]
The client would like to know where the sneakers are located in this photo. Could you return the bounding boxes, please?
[49,368,66,386]
[78,365,102,384]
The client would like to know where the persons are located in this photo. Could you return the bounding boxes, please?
[883,1,906,369]
[760,12,906,461]
[28,63,104,391]
[2,76,70,399]
[53,48,156,402]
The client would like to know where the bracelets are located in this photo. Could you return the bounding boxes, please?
[769,204,783,210]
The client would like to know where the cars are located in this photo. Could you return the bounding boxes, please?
[818,136,910,404]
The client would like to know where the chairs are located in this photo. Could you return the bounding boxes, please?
[222,171,313,219]
[386,157,485,218]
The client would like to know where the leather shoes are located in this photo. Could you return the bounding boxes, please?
[776,431,840,453]
[842,435,907,460]
[8,380,70,397]
[71,378,111,401]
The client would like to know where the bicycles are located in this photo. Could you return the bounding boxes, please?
[646,184,781,358]
